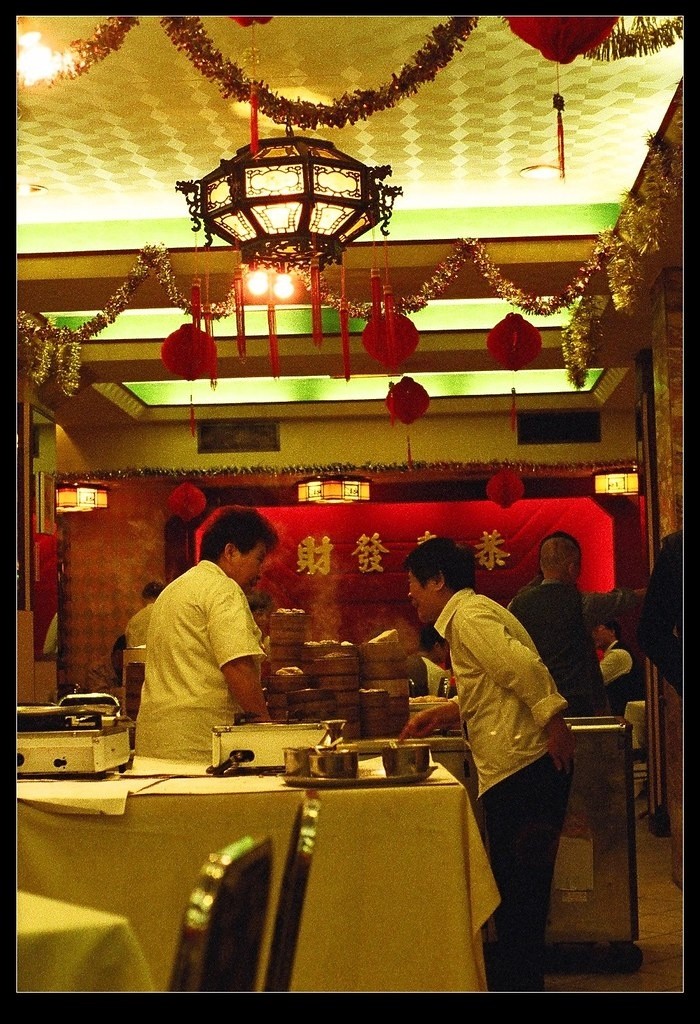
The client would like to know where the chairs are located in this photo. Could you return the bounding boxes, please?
[624,701,648,799]
[168,797,322,992]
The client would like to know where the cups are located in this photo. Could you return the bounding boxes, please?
[308,751,358,779]
[283,747,314,776]
[382,744,431,776]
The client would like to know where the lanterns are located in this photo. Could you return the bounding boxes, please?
[384,376,430,472]
[502,17,622,181]
[161,324,217,437]
[486,312,542,433]
[362,314,420,427]
[164,483,207,561]
[230,16,272,154]
[486,467,525,508]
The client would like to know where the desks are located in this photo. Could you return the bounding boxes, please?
[16,753,500,992]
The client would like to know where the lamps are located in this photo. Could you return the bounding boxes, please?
[55,484,108,513]
[299,478,373,505]
[593,471,640,494]
[175,119,405,276]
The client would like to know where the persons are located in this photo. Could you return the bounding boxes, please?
[112,509,278,761]
[399,530,683,992]
